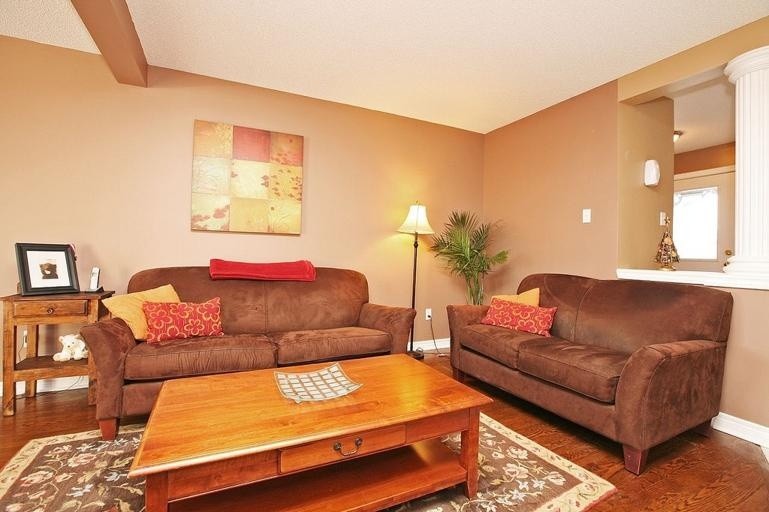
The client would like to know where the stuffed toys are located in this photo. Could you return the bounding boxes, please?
[52,333,88,362]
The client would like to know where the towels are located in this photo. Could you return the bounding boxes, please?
[209,259,316,282]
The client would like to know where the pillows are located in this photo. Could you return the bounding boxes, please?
[480,298,558,338]
[101,283,181,342]
[143,297,224,344]
[490,287,540,307]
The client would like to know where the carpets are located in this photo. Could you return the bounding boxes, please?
[0,410,618,512]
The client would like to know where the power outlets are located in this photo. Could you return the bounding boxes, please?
[425,308,432,320]
[23,330,27,347]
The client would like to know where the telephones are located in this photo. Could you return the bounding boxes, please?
[86,267,104,293]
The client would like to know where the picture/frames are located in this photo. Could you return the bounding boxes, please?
[14,242,80,295]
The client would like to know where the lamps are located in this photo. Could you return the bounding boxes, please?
[396,200,434,361]
[672,130,683,142]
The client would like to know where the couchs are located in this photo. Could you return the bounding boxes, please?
[81,267,416,440]
[446,273,734,476]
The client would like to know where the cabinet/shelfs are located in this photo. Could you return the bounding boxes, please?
[0,291,115,416]
